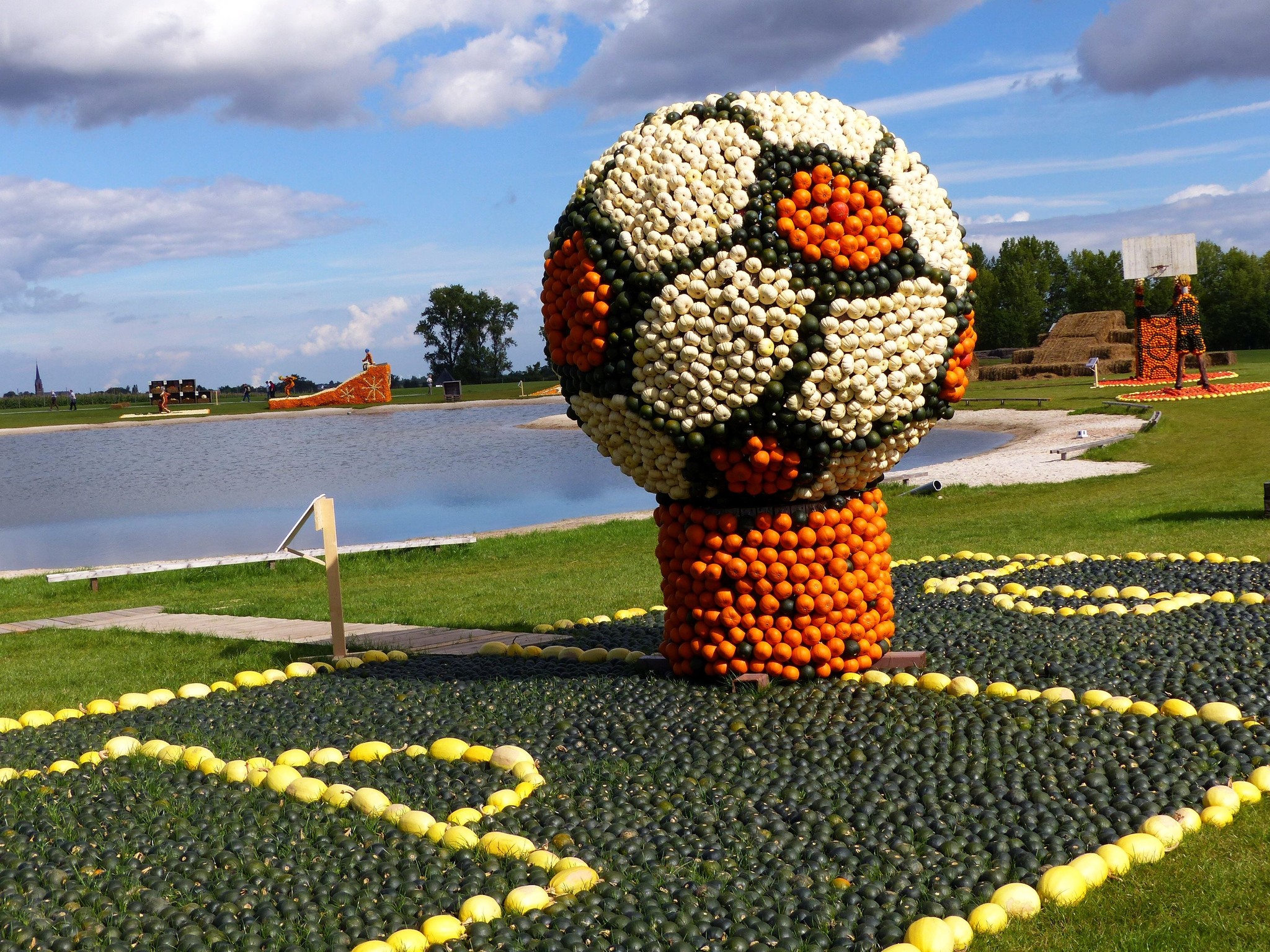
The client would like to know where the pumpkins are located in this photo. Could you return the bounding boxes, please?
[539,91,979,681]
[1134,275,1206,380]
[0,549,1270,952]
[1092,371,1239,387]
[1115,381,1270,403]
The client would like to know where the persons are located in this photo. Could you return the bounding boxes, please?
[427,374,433,395]
[279,375,297,398]
[1172,274,1210,387]
[68,390,77,410]
[266,381,275,398]
[49,391,59,411]
[362,349,373,370]
[158,386,171,413]
[241,384,251,402]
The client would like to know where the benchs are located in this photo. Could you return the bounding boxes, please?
[1092,400,1153,412]
[1049,432,1136,461]
[879,469,932,487]
[1148,409,1163,426]
[961,397,1053,406]
[44,534,478,593]
